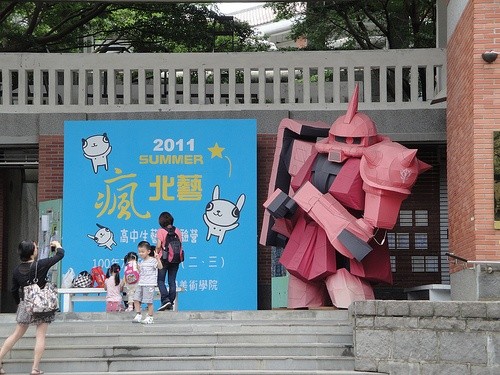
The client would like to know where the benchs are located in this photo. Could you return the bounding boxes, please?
[54,287,184,312]
[403,284,450,301]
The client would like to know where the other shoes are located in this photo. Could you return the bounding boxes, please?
[30,368,45,375]
[0,366,7,374]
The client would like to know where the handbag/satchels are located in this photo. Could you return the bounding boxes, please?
[24,259,61,315]
[92,266,106,288]
[72,271,93,288]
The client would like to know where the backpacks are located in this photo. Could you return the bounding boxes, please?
[163,226,185,265]
[125,263,140,285]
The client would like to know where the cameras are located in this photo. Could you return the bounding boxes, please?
[51,245,56,252]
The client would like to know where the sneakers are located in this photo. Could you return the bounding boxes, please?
[157,300,171,311]
[142,315,154,324]
[132,312,142,323]
